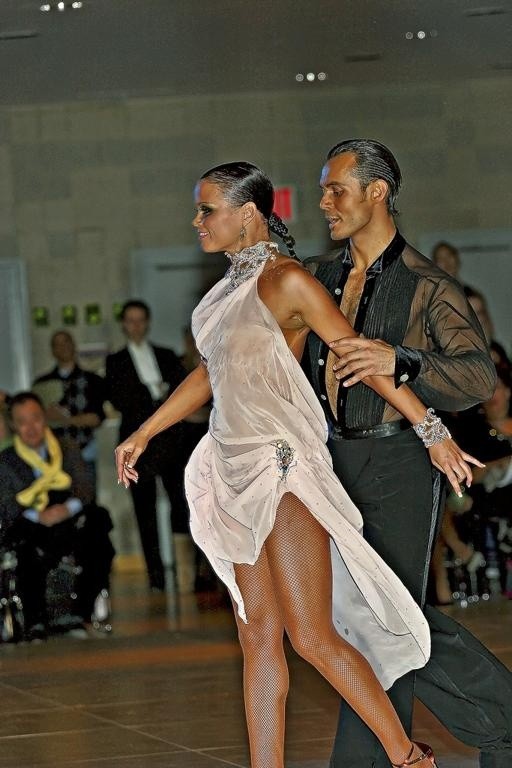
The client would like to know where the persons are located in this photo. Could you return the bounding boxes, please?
[1,391,118,642]
[431,240,512,607]
[105,300,229,615]
[115,161,488,768]
[301,138,512,768]
[32,329,107,499]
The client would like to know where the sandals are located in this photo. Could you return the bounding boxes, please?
[391,741,439,768]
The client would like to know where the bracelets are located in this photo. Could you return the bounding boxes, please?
[412,408,453,448]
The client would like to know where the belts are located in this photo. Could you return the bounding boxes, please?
[328,418,412,442]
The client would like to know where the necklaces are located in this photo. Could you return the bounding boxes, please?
[225,246,275,294]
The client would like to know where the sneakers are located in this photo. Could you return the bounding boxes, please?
[29,619,87,646]
